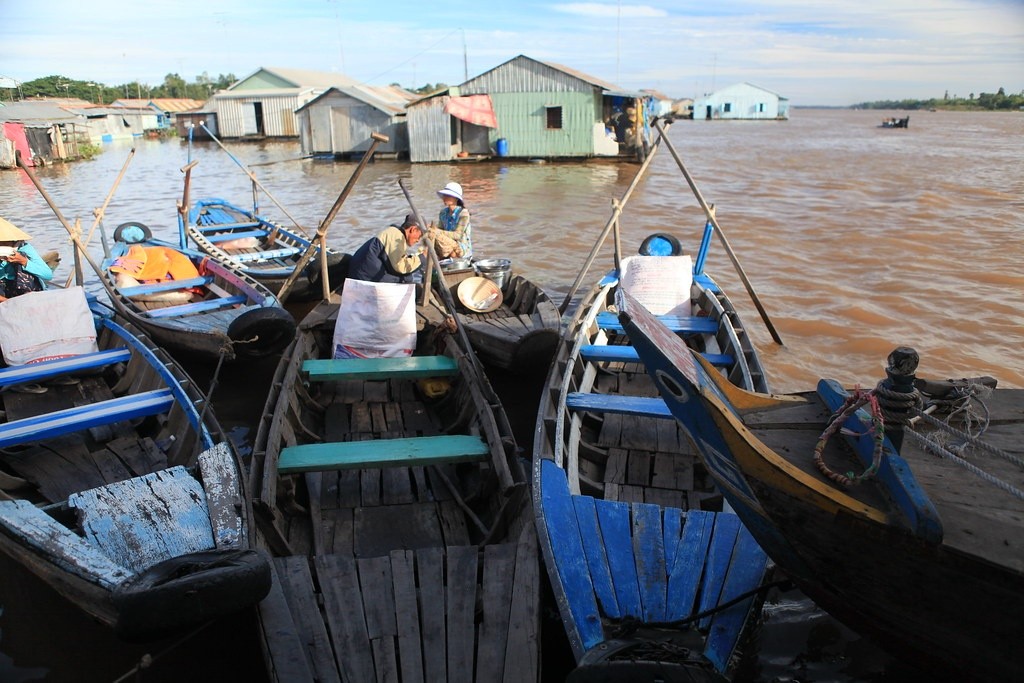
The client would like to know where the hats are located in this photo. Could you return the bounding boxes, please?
[437,182,463,203]
[404,214,428,236]
[0,216,33,241]
[457,276,504,313]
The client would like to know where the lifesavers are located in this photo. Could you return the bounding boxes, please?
[225,305,297,361]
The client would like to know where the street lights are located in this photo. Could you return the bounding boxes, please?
[86,83,96,104]
[63,83,71,97]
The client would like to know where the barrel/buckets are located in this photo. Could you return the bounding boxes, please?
[439,258,470,271]
[496,138,507,156]
[477,259,512,294]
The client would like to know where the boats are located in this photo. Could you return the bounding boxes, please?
[877,115,909,129]
[613,279,1024,683]
[398,249,564,370]
[241,263,547,683]
[529,233,778,683]
[95,218,298,363]
[181,195,334,295]
[0,220,273,633]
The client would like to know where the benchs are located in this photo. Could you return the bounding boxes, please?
[0,345,131,386]
[198,221,260,231]
[275,433,492,473]
[117,275,214,296]
[206,228,270,242]
[232,245,303,261]
[566,391,676,419]
[301,355,460,380]
[0,386,176,448]
[597,311,718,333]
[146,294,247,318]
[580,343,735,368]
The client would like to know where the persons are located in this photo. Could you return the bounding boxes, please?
[346,214,428,283]
[427,182,472,266]
[0,217,52,298]
